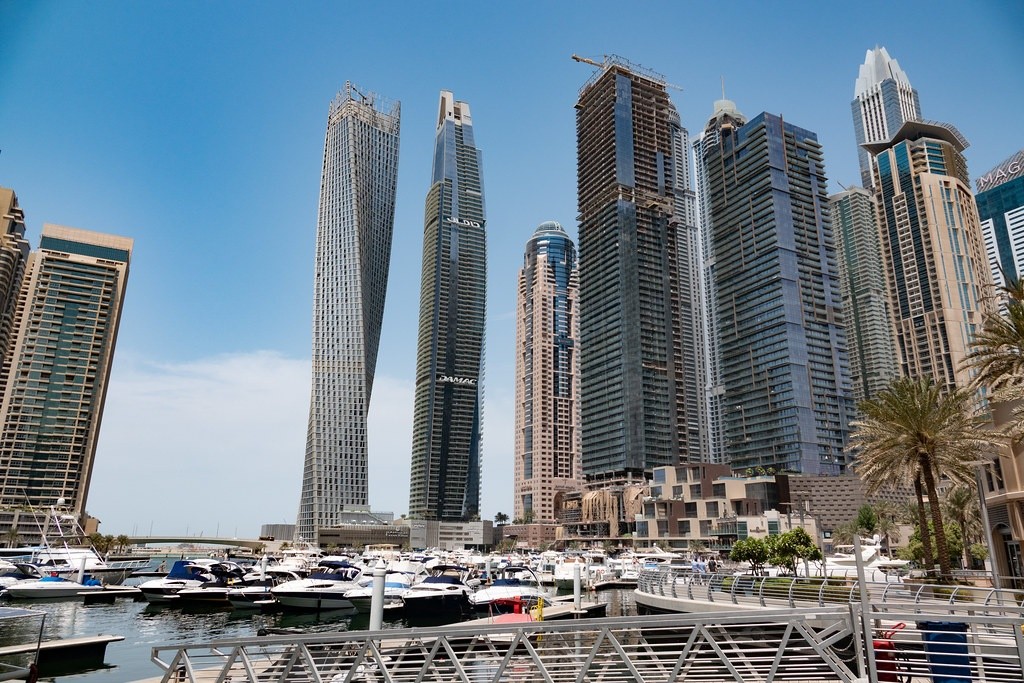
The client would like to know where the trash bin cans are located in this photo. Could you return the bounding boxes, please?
[916,620,972,683]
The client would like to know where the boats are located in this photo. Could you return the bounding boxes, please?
[0,536,694,654]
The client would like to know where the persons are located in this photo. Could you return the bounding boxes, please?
[691,556,717,585]
